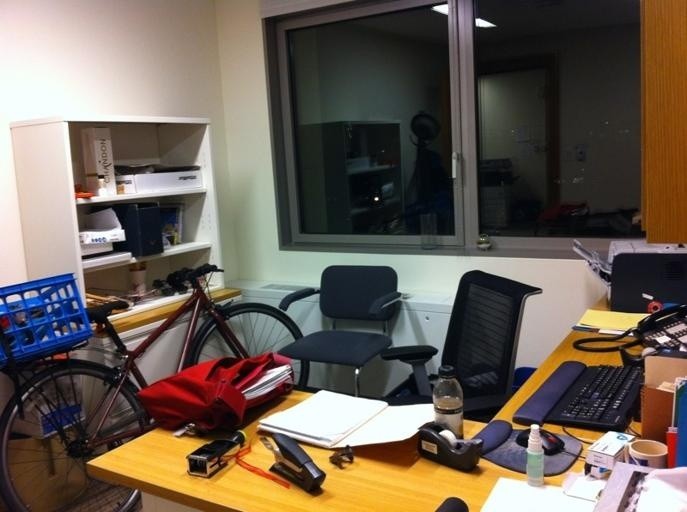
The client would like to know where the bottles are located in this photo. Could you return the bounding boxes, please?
[431,365,465,441]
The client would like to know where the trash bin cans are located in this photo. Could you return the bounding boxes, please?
[478,175,513,230]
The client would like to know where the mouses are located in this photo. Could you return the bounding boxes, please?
[516,427,565,456]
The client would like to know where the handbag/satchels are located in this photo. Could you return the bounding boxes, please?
[135,351,296,435]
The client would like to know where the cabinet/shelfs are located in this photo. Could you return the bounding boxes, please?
[12,117,225,330]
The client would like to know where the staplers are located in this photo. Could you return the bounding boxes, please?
[269,433,326,492]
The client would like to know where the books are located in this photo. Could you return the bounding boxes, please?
[159,202,186,244]
[262,384,439,450]
[233,361,292,405]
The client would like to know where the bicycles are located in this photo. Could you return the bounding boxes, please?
[2,263,310,512]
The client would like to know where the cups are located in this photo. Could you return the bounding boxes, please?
[624,439,670,470]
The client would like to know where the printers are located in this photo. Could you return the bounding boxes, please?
[571,239,687,313]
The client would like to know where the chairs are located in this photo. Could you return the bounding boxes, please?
[382,271,543,420]
[279,267,410,398]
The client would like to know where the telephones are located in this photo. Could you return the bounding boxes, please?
[631,305,687,355]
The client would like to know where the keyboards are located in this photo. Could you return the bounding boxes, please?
[544,365,644,430]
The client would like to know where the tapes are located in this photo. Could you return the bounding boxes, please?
[438,429,483,449]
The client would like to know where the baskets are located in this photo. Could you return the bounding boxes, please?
[0,273,93,366]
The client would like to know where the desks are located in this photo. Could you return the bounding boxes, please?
[86,292,687,510]
